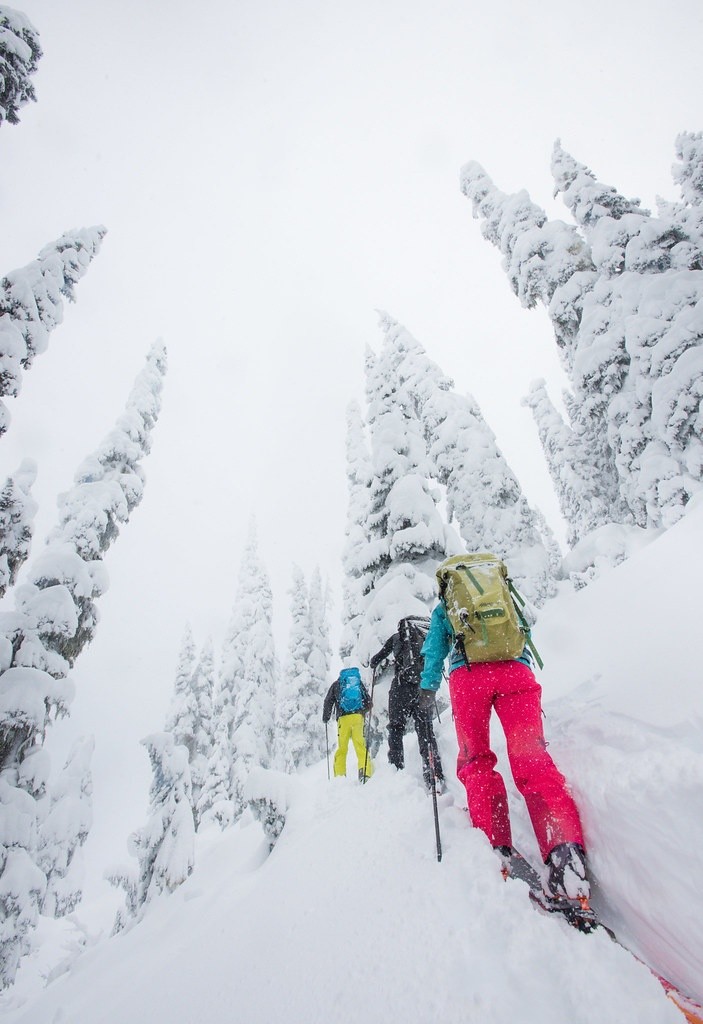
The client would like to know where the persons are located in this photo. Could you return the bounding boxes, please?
[418,588,592,901]
[323,676,373,782]
[371,620,445,795]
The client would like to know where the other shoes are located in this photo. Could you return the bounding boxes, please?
[391,763,406,775]
[359,775,369,784]
[546,841,591,895]
[492,845,512,868]
[428,775,445,797]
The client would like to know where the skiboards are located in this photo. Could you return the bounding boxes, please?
[491,844,553,925]
[554,893,703,1024]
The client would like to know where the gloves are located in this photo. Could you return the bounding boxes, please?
[406,688,437,723]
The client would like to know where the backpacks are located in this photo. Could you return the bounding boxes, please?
[435,551,527,664]
[337,666,366,714]
[395,616,446,686]
